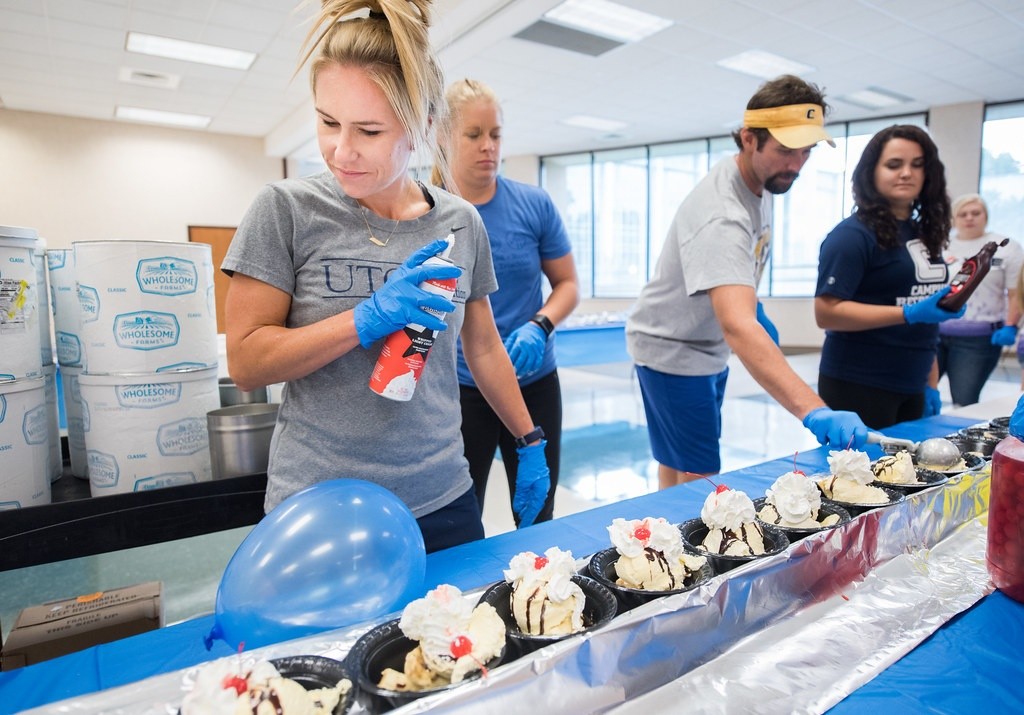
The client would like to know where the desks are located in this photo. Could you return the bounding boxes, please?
[0,416,1024,715]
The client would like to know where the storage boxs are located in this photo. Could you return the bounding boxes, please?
[0,580,164,672]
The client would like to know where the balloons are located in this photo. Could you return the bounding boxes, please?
[203,480,425,653]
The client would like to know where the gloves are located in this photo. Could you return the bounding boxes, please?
[803,407,876,450]
[756,303,778,346]
[991,325,1017,345]
[354,239,462,351]
[903,287,967,325]
[512,440,550,529]
[920,385,940,417]
[504,322,545,381]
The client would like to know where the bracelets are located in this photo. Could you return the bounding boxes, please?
[514,426,545,448]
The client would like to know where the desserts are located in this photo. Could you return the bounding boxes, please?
[174,441,969,715]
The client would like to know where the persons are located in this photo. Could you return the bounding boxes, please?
[936,192,1024,407]
[625,75,886,492]
[220,0,550,554]
[814,124,967,430]
[429,78,581,530]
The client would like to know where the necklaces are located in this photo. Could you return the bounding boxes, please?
[356,178,412,247]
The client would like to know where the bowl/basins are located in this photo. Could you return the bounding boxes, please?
[889,451,984,481]
[868,462,946,498]
[677,519,790,576]
[474,575,617,656]
[344,619,506,710]
[812,485,905,519]
[207,404,280,480]
[218,376,268,407]
[946,417,1013,462]
[750,495,850,544]
[177,656,355,714]
[590,547,716,617]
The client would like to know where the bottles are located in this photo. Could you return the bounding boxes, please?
[370,233,457,401]
[935,238,1010,313]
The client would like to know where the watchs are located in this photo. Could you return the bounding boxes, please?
[527,315,554,336]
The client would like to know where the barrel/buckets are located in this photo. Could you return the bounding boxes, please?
[42,363,62,481]
[0,226,43,384]
[45,249,82,366]
[0,374,51,509]
[35,254,55,365]
[58,360,89,477]
[77,363,221,498]
[72,241,216,375]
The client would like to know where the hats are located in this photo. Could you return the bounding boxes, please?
[745,104,837,149]
